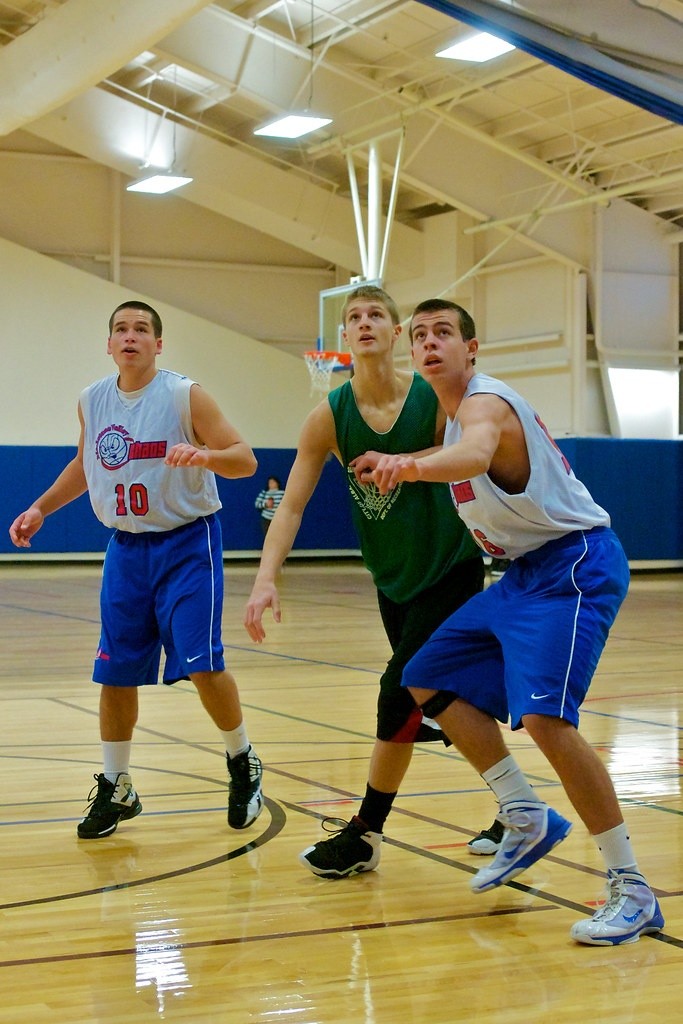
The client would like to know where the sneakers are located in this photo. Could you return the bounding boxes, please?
[77,772,142,839]
[225,744,264,829]
[470,803,573,894]
[467,800,506,855]
[299,815,384,880]
[571,863,665,945]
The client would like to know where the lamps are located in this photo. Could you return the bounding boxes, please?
[252,0,332,140]
[434,22,517,62]
[124,65,193,194]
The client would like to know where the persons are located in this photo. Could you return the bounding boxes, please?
[242,285,534,882]
[369,296,666,948]
[9,300,265,841]
